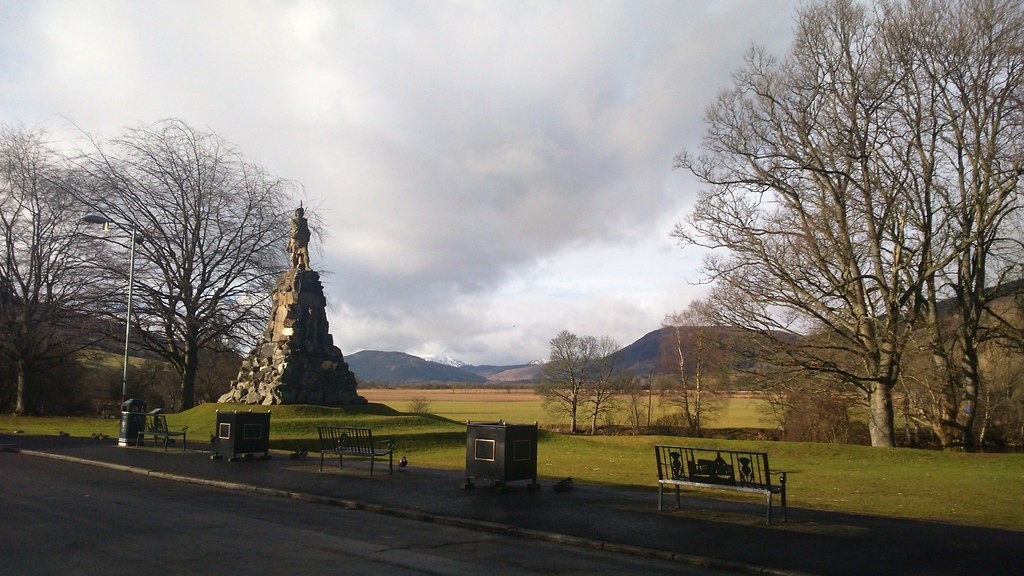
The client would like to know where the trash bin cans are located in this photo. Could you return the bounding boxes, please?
[117,395,148,448]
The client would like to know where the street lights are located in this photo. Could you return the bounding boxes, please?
[81,215,145,403]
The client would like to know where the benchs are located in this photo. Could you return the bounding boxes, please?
[130,413,188,452]
[655,445,787,526]
[313,427,393,477]
